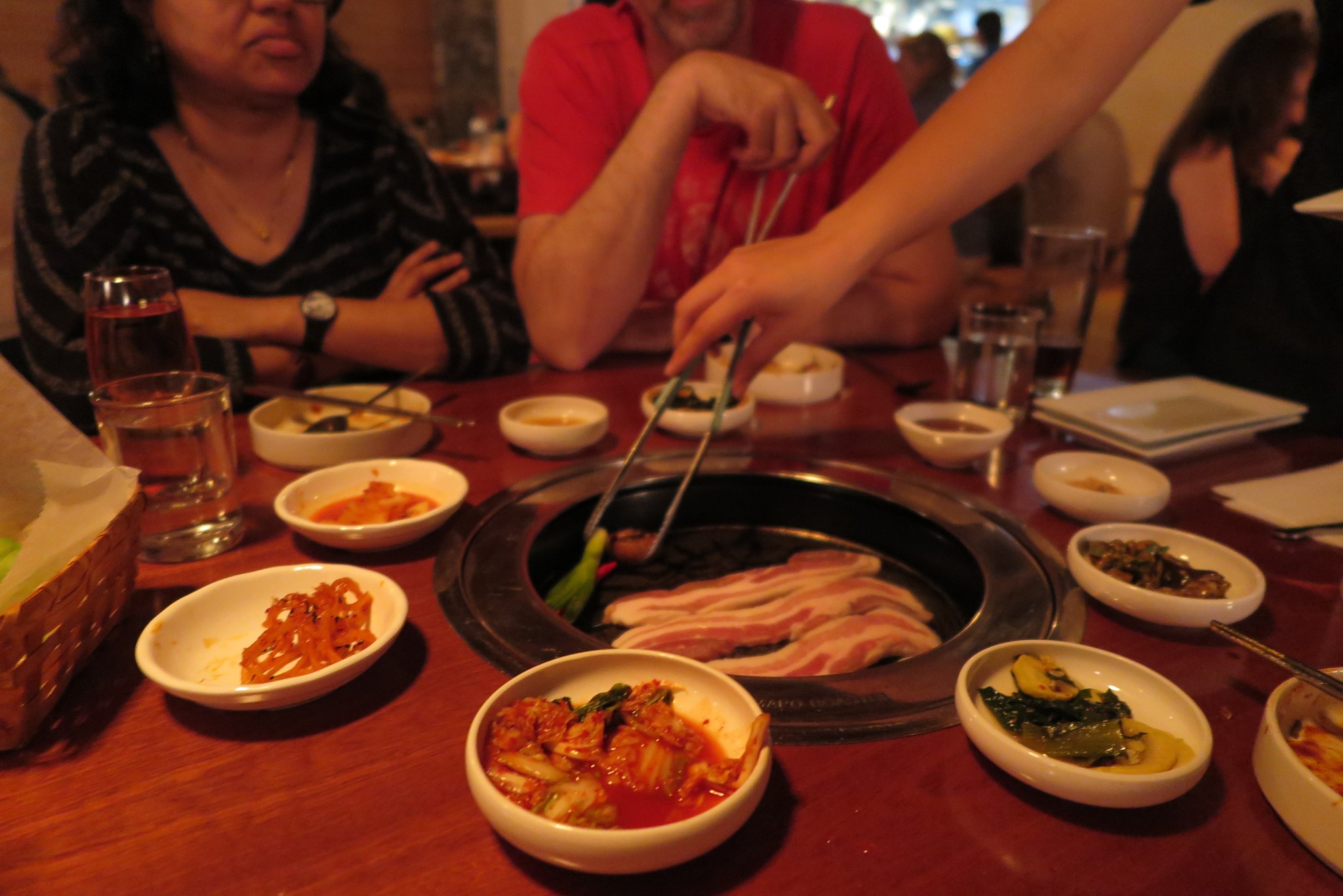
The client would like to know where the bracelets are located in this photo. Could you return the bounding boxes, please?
[294,357,318,389]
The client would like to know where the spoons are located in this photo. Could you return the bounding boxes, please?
[302,367,427,434]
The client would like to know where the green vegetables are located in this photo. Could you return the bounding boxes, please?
[542,529,608,624]
[979,684,1149,768]
[555,679,673,732]
[1092,539,1169,563]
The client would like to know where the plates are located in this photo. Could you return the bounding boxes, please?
[1031,377,1308,463]
[1294,189,1343,220]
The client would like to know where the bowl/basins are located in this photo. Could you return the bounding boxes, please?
[893,400,1013,471]
[458,451,1055,723]
[499,395,609,457]
[1250,668,1343,879]
[955,640,1215,810]
[1066,522,1266,630]
[464,648,774,874]
[248,384,432,471]
[135,563,410,711]
[640,381,757,436]
[705,344,844,403]
[273,457,468,551]
[1033,450,1172,524]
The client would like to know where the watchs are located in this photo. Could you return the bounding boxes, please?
[300,291,337,355]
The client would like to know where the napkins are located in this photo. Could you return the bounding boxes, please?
[1210,459,1343,554]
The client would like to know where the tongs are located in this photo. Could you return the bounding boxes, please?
[580,317,752,560]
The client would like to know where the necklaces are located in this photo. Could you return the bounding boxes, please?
[201,165,293,242]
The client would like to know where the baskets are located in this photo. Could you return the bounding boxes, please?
[0,468,146,758]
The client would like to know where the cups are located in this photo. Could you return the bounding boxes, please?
[1018,225,1108,397]
[88,368,246,569]
[955,300,1046,424]
[82,266,200,404]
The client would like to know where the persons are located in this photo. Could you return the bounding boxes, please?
[664,1,1342,465]
[967,11,1004,75]
[512,1,964,372]
[15,1,530,434]
[894,33,957,128]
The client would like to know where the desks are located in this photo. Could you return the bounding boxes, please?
[1,352,1343,892]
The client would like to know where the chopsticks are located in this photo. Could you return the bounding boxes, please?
[742,96,835,246]
[1209,619,1343,700]
